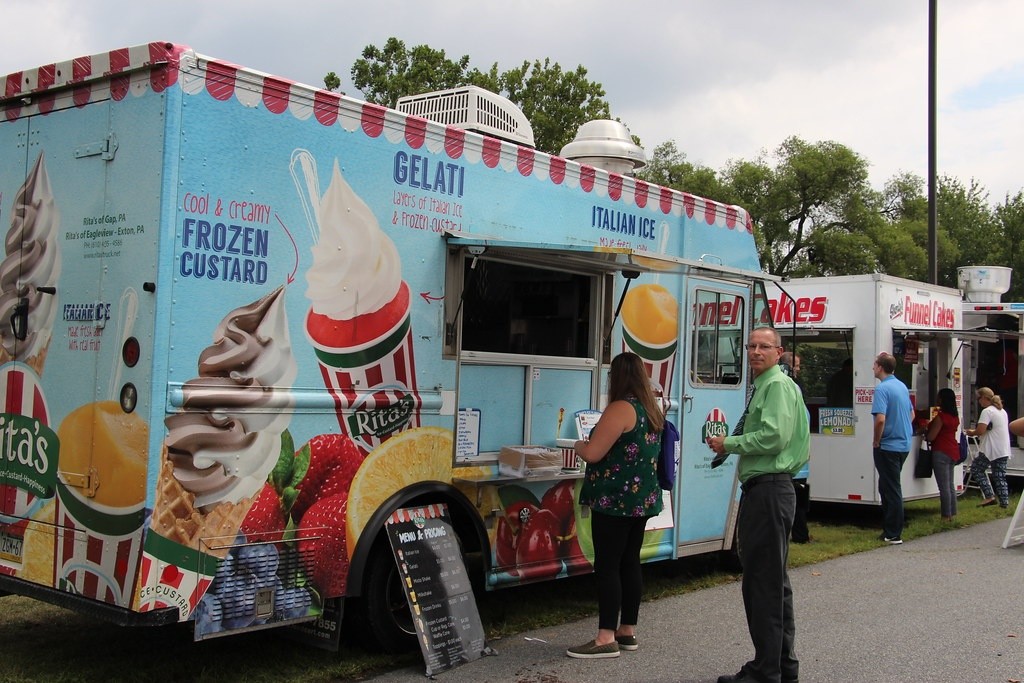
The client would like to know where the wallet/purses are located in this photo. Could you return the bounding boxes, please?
[975,422,992,430]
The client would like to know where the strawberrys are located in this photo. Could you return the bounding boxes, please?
[233,432,362,600]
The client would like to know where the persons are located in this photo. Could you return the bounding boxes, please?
[565,350,667,660]
[708,325,811,683]
[924,387,961,526]
[1009,417,1024,437]
[778,349,811,545]
[827,358,853,408]
[963,387,1011,509]
[871,352,916,545]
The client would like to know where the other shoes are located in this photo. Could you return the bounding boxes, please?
[976,497,998,508]
[876,535,903,544]
[717,659,799,683]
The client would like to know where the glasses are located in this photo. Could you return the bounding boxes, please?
[748,342,779,350]
[608,372,611,375]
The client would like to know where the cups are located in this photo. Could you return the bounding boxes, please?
[561,448,581,473]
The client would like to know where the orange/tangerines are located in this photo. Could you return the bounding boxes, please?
[22,501,55,589]
[346,426,500,560]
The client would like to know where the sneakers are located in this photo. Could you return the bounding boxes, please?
[566,639,620,659]
[615,635,638,651]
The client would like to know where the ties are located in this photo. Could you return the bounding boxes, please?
[711,386,756,470]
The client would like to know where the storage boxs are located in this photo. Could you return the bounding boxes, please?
[555,446,581,471]
[908,389,917,419]
[498,445,560,479]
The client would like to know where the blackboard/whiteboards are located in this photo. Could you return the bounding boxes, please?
[1007,489,1024,540]
[457,408,481,456]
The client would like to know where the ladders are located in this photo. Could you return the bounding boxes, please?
[956,435,995,499]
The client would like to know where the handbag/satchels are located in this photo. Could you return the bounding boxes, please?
[955,424,968,465]
[914,441,933,478]
[658,419,679,491]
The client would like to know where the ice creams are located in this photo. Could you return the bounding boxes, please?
[305,156,420,455]
[136,282,298,624]
[0,149,64,379]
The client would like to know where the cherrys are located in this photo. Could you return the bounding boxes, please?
[495,480,591,584]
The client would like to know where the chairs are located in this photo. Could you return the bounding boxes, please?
[956,434,994,500]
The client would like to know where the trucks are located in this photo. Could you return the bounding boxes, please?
[0,44,808,671]
